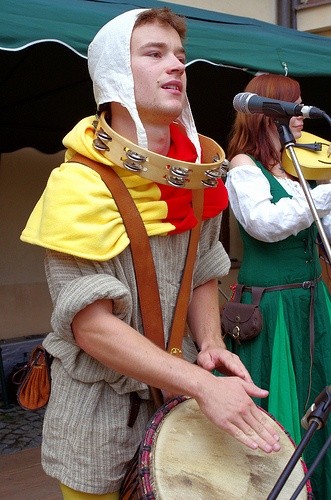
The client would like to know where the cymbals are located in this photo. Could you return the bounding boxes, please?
[93,108,230,190]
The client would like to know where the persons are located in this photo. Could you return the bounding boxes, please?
[34,0,284,498]
[227,71,329,469]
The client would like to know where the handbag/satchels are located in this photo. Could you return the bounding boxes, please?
[222,302,262,340]
[12,345,52,412]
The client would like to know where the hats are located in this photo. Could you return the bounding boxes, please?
[87,8,201,165]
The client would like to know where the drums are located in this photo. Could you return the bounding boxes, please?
[116,393,314,500]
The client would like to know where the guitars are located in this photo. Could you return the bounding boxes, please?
[280,130,331,180]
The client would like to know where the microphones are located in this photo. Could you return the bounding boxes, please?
[233,91,324,119]
[300,385,331,431]
